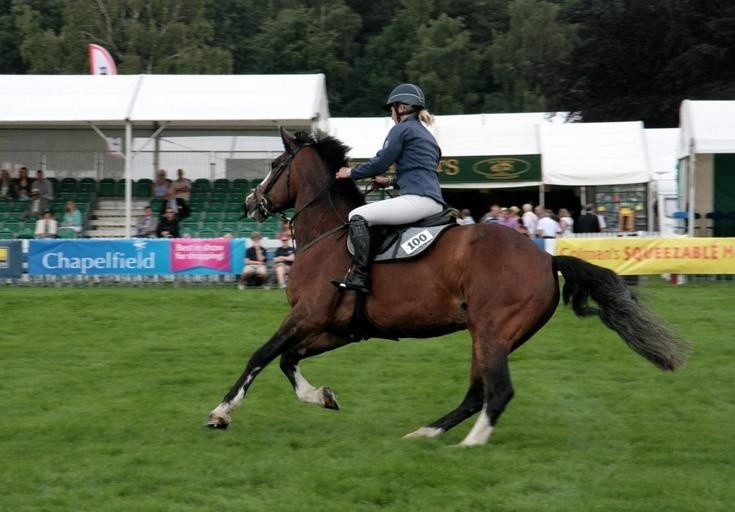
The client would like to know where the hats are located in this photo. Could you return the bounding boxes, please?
[597,205,606,212]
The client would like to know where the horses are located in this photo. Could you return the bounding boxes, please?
[204,124,694,453]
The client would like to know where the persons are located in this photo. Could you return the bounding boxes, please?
[277,216,295,241]
[133,204,158,239]
[0,169,14,201]
[238,231,269,289]
[272,238,296,288]
[151,169,169,200]
[12,166,33,202]
[330,82,447,296]
[455,200,609,236]
[56,199,83,233]
[31,168,54,200]
[169,168,192,201]
[21,188,49,220]
[159,190,190,220]
[156,207,179,238]
[33,209,59,237]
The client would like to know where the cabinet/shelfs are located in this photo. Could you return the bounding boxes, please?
[592,183,648,231]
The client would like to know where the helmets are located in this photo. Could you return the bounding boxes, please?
[386,83,425,108]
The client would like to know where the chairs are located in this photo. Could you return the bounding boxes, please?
[0,177,296,240]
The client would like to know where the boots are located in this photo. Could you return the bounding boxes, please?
[330,214,373,294]
